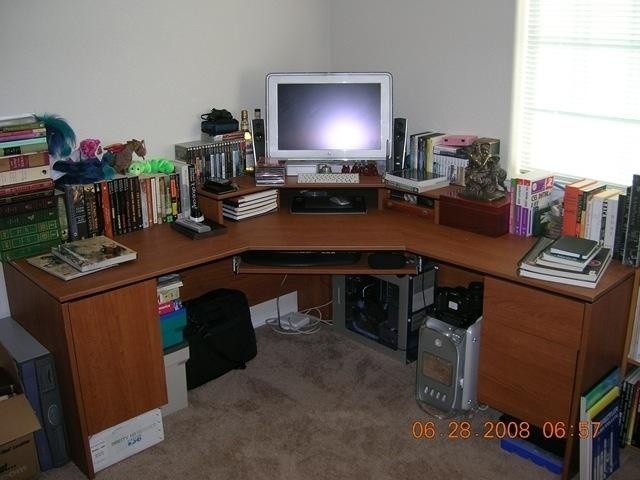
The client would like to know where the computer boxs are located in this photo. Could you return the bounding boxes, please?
[415,309,483,420]
[331,266,437,366]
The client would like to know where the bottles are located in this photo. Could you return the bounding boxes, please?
[241,110,249,132]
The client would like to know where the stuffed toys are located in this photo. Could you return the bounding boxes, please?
[35,115,175,191]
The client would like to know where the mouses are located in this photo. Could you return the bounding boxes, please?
[328,195,353,206]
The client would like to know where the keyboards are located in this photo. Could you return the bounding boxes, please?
[241,251,361,267]
[297,173,360,184]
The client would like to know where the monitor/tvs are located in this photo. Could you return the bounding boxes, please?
[264,71,394,176]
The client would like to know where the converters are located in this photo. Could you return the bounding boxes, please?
[277,312,311,330]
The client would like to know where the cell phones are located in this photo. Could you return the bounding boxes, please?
[209,177,232,186]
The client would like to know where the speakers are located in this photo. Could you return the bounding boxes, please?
[251,119,265,168]
[393,117,408,171]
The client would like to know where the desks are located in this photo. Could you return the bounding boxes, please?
[2,174,639,480]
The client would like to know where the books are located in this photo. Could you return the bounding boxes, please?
[51,235,137,273]
[384,131,470,194]
[222,189,279,221]
[508,170,640,289]
[171,215,228,241]
[201,132,244,143]
[579,287,640,480]
[1,189,69,262]
[26,252,118,280]
[62,160,199,242]
[255,163,284,186]
[2,114,54,204]
[175,141,254,187]
[157,280,183,316]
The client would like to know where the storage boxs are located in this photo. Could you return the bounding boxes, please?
[439,187,510,237]
[160,342,189,417]
[160,307,187,348]
[0,392,42,480]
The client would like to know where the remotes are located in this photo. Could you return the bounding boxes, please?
[175,218,211,234]
